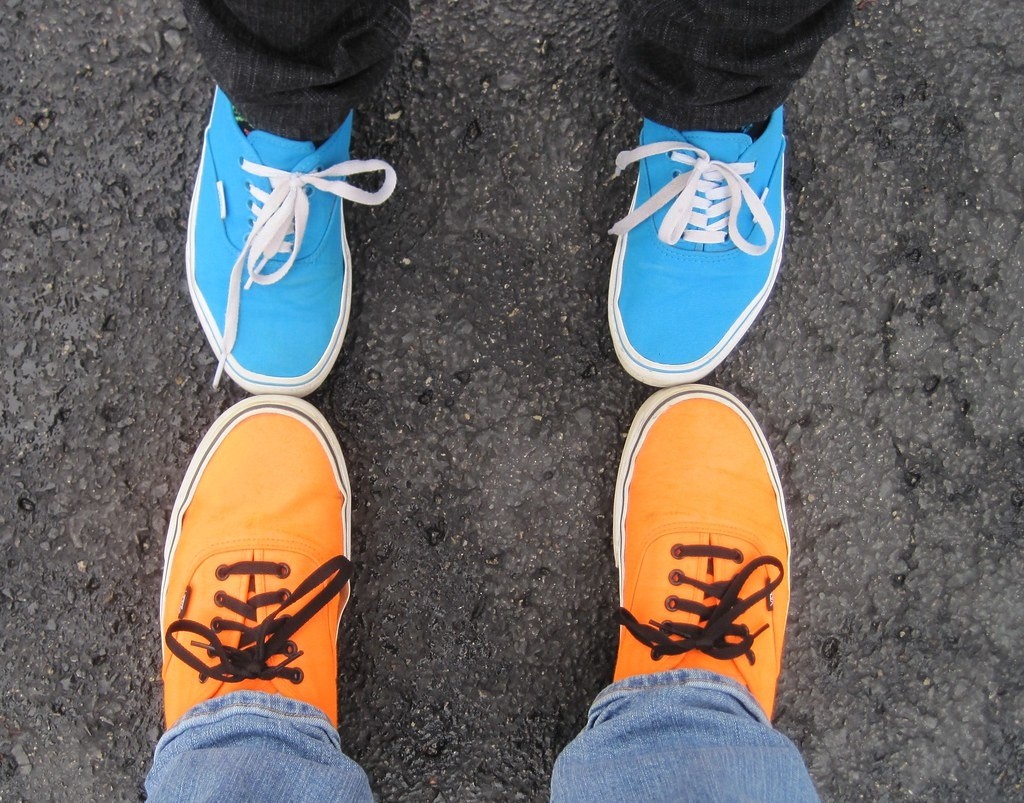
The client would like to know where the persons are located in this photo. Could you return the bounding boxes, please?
[132,384,821,803]
[183,1,856,399]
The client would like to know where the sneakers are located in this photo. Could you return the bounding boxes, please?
[613,382,790,727]
[609,104,786,384]
[161,394,354,729]
[186,83,352,399]
